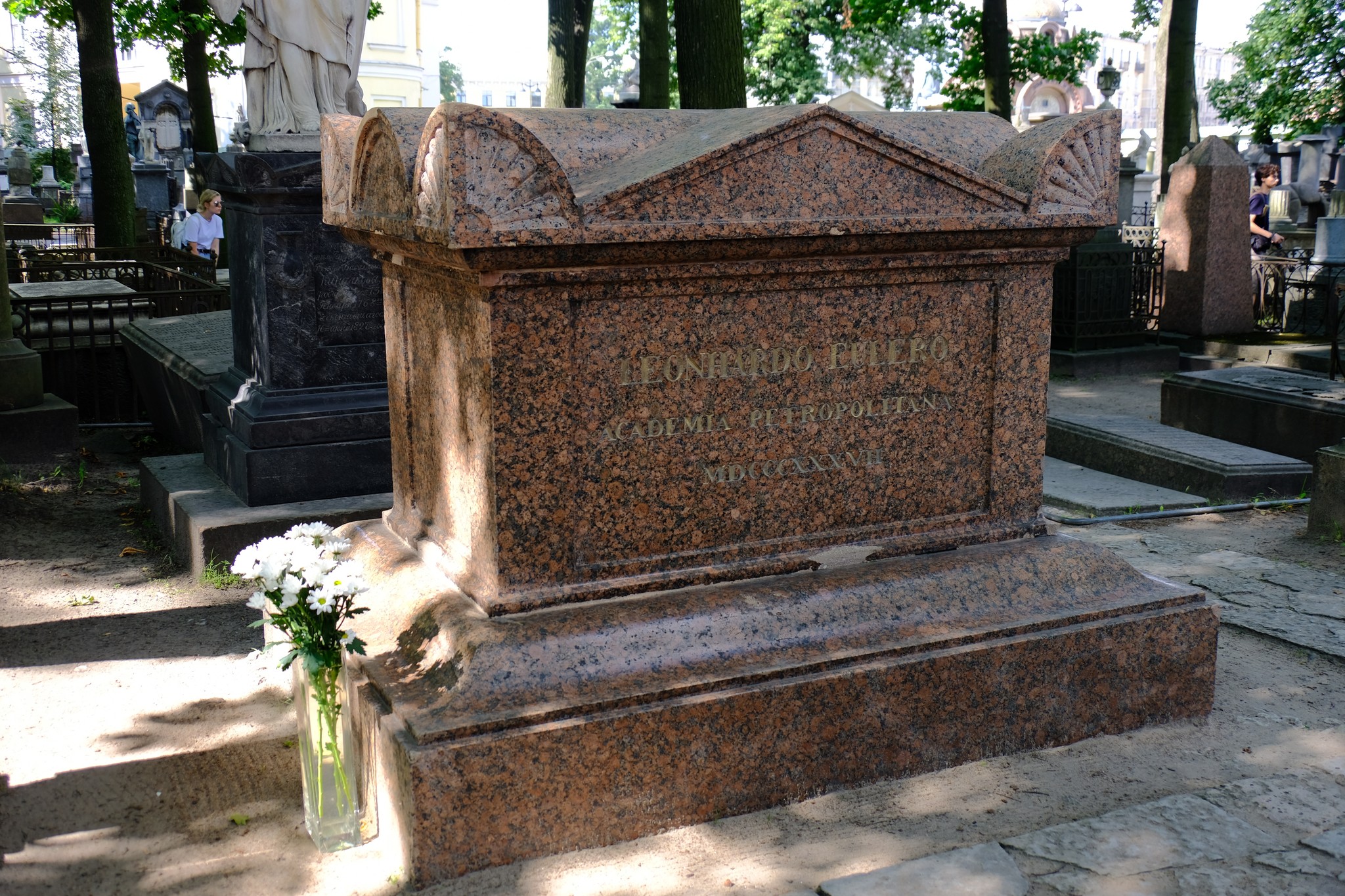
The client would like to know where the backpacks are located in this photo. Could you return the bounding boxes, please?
[170,217,190,250]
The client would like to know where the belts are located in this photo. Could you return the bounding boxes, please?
[196,249,211,254]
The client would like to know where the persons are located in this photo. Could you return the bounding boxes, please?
[172,203,191,224]
[1127,130,1151,159]
[1249,162,1286,319]
[171,188,224,266]
[123,102,143,164]
[206,0,370,137]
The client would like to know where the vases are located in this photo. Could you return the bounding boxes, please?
[291,650,362,854]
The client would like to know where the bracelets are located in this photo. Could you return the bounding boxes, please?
[1269,230,1276,241]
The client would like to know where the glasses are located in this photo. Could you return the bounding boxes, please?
[214,201,222,207]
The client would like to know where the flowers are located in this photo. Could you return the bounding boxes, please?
[229,521,372,819]
